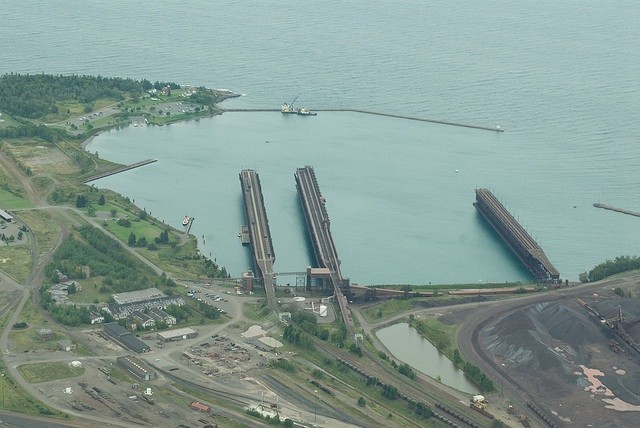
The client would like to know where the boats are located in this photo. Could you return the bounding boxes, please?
[282,105,296,113]
[297,108,316,114]
